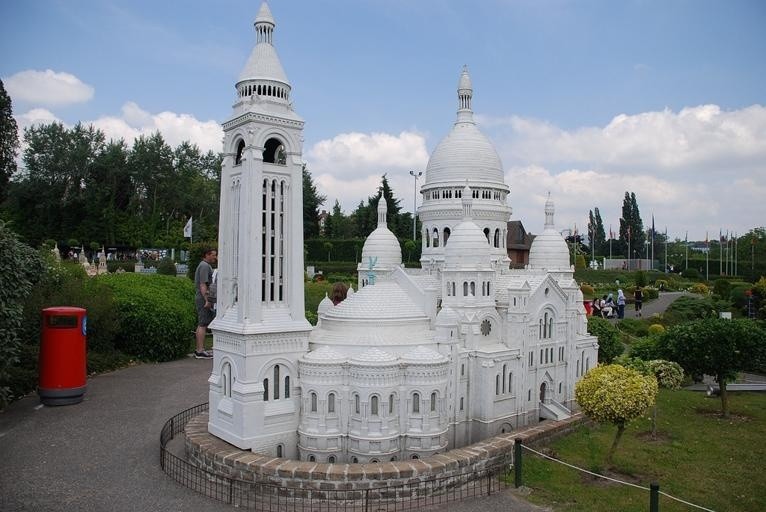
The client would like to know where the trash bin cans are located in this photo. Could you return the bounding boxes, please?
[41,306,87,405]
[583,300,593,315]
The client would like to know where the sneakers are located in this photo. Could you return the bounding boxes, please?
[193,350,213,360]
[607,316,615,319]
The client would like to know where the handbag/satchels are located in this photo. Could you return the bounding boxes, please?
[619,297,626,301]
[206,273,217,303]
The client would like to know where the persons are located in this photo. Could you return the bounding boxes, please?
[590,289,626,319]
[212,255,218,316]
[193,248,217,359]
[615,278,620,287]
[331,283,347,305]
[115,264,125,272]
[622,261,627,269]
[633,287,644,317]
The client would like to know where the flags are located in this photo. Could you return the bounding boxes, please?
[184,217,192,237]
[574,216,737,245]
